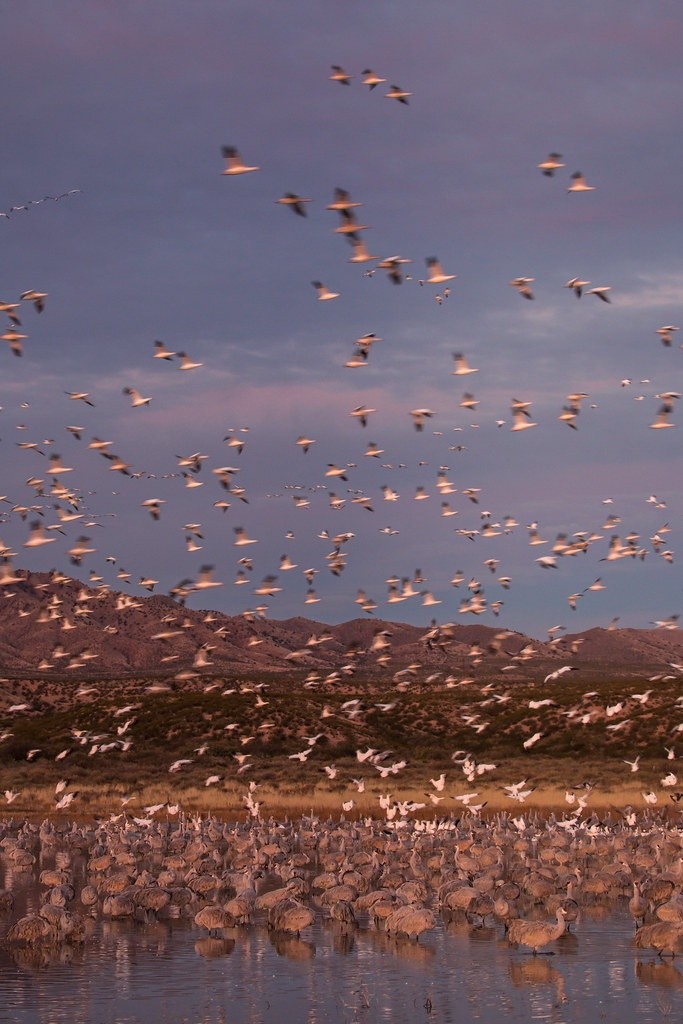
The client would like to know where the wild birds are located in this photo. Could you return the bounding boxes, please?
[506,906,570,959]
[0,59,683,832]
[508,953,568,1007]
[4,913,58,950]
[0,809,683,937]
[195,936,235,962]
[634,919,683,960]
